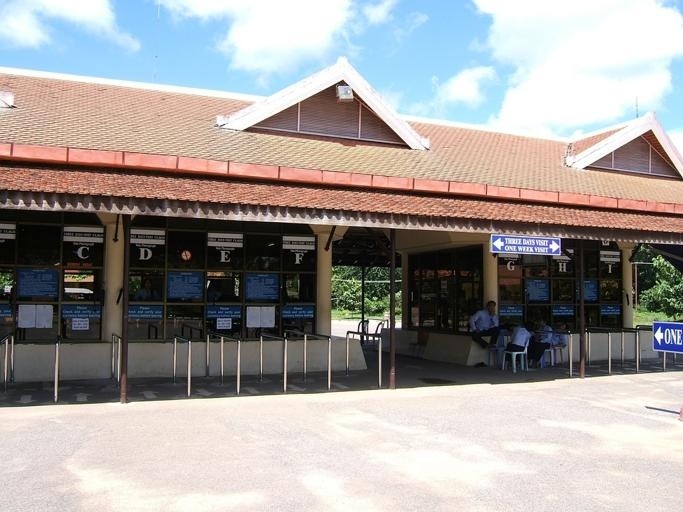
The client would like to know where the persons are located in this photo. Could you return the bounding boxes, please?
[468,301,500,348]
[504,324,534,363]
[529,317,553,368]
[133,278,159,302]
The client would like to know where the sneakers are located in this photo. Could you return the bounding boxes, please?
[477,361,487,368]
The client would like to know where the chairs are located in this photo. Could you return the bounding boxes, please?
[488,331,566,373]
